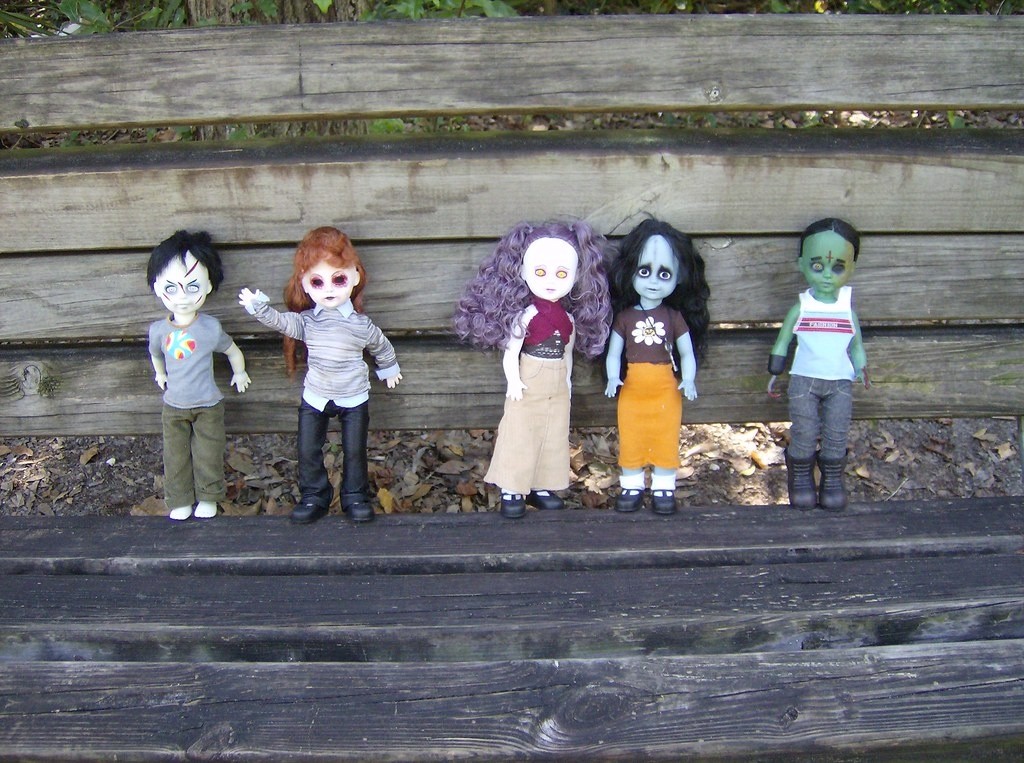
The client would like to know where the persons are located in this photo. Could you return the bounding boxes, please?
[766,218,871,512]
[237,226,405,525]
[145,229,252,521]
[603,219,711,516]
[453,219,614,518]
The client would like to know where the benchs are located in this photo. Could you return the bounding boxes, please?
[0,13,1024,763]
